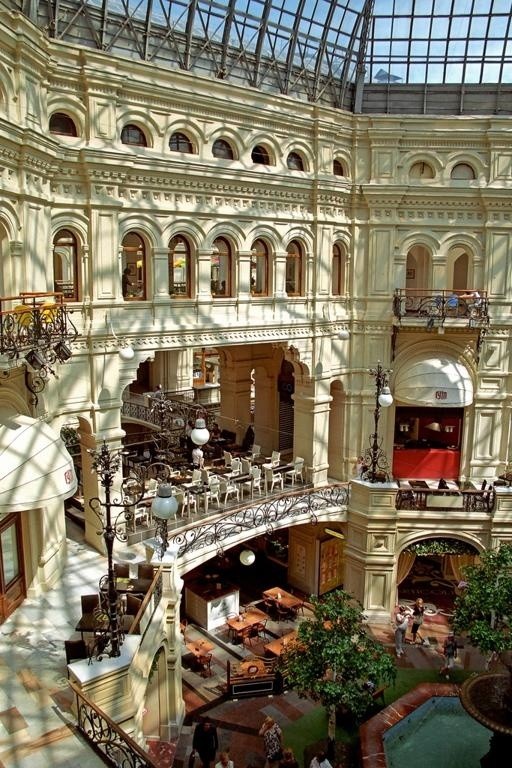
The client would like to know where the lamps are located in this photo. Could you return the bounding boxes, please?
[424,318,476,334]
[25,341,73,372]
[336,313,350,340]
[359,364,395,486]
[238,540,258,565]
[106,310,136,361]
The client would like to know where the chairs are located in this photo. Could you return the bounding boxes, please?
[186,585,312,678]
[426,294,483,318]
[64,561,161,680]
[121,444,306,532]
[393,469,511,513]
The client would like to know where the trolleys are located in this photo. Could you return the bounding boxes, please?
[416,631,439,648]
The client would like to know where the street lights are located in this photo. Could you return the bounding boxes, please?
[354,357,397,485]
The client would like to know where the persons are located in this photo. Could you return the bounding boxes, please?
[148,385,162,412]
[258,716,283,767]
[463,290,482,315]
[409,597,430,646]
[439,634,458,680]
[121,267,133,296]
[185,420,195,436]
[395,604,413,658]
[210,423,220,438]
[457,291,477,312]
[351,455,370,480]
[309,750,334,768]
[214,752,234,767]
[188,716,218,767]
[279,748,299,767]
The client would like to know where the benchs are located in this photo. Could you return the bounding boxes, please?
[335,687,386,728]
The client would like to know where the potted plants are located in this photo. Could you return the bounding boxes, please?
[443,539,512,768]
[273,586,400,767]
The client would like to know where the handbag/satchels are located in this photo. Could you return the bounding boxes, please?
[390,623,397,632]
[187,751,196,768]
